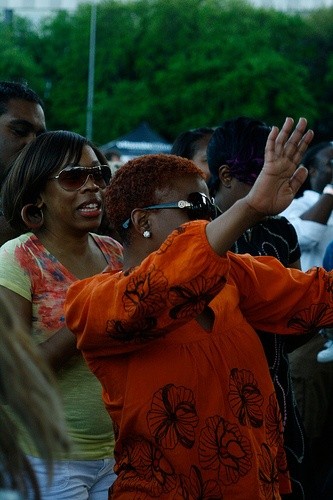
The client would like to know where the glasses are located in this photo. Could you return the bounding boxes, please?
[122,192,218,229]
[46,165,111,192]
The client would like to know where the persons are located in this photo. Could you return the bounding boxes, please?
[104,125,333,500]
[66,115,333,500]
[0,78,48,250]
[0,294,73,500]
[0,129,124,500]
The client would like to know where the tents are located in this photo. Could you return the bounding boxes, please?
[96,119,175,164]
